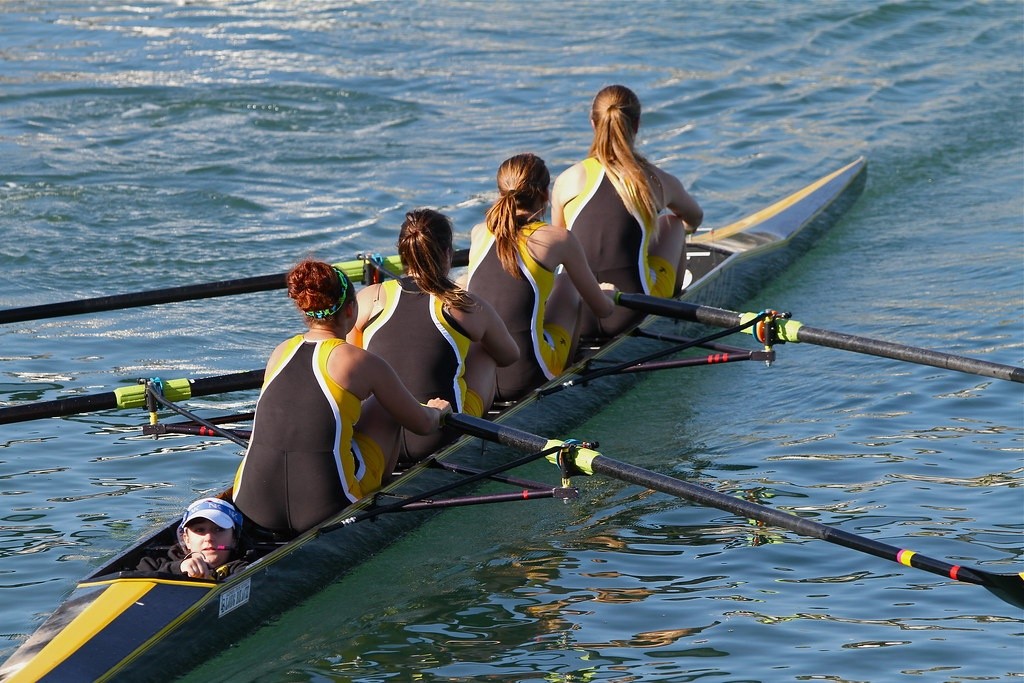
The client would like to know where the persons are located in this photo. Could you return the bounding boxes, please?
[454,153,620,404]
[345,209,521,455]
[136,498,251,581]
[550,84,705,330]
[232,259,454,539]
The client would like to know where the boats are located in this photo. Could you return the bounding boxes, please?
[0,153,868,683]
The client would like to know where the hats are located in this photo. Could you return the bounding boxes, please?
[181,498,236,530]
